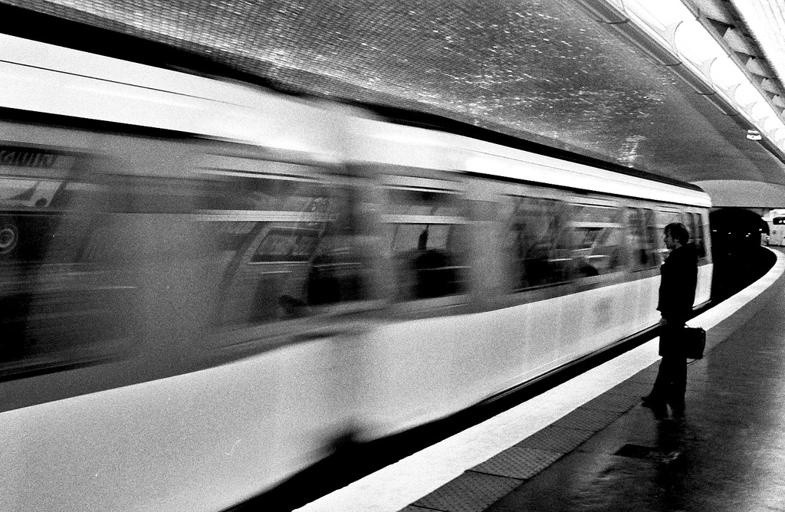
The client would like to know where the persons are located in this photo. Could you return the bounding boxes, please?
[638,223,699,411]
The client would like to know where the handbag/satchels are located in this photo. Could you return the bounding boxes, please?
[659,327,705,359]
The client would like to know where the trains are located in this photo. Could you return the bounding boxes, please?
[0,31,715,510]
